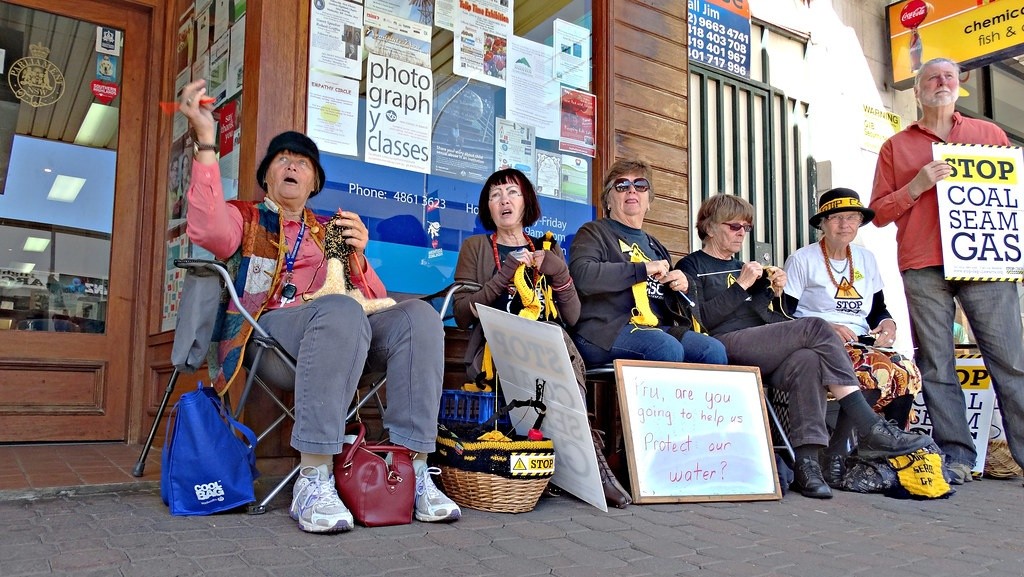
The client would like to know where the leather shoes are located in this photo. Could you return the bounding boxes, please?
[826,450,845,487]
[792,457,832,499]
[854,415,934,458]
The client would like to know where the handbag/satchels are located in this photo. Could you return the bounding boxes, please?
[332,422,415,528]
[841,428,948,494]
[160,380,258,515]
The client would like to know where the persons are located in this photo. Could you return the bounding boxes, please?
[869,57,1024,488]
[782,187,922,486]
[68,278,89,294]
[178,78,463,534]
[346,44,357,59]
[170,155,191,217]
[568,156,794,498]
[346,25,360,43]
[674,194,934,498]
[454,169,632,509]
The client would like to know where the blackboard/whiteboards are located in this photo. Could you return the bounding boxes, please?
[614,357,782,504]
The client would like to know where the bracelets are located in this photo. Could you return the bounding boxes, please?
[550,276,573,291]
[193,141,218,155]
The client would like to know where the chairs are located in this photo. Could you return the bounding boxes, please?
[588,364,795,462]
[173,258,482,516]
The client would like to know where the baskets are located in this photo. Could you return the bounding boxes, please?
[434,422,556,514]
[438,388,510,426]
[985,439,1023,478]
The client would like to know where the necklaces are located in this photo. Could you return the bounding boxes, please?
[492,232,536,293]
[829,259,848,274]
[821,235,854,290]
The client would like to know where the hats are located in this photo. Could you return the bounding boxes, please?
[254,131,326,199]
[809,188,875,231]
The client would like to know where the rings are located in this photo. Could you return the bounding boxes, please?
[675,282,678,285]
[889,339,893,343]
[884,331,888,335]
[516,247,523,252]
[187,99,192,105]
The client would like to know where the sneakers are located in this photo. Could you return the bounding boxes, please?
[411,460,461,522]
[942,459,972,485]
[290,464,354,533]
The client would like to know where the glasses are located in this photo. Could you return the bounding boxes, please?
[605,177,650,197]
[719,219,754,232]
[824,213,865,223]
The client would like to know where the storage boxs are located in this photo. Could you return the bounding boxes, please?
[439,388,509,425]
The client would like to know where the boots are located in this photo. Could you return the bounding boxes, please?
[588,421,632,509]
